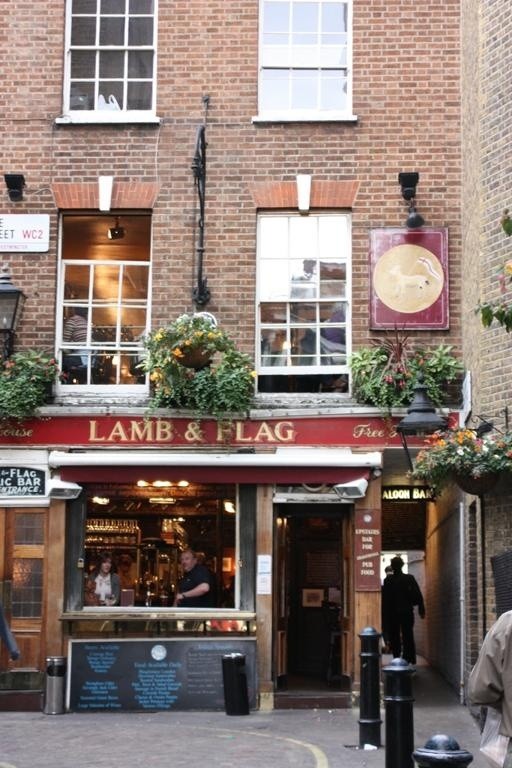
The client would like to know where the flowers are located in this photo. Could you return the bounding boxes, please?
[409,430,512,495]
[141,316,256,420]
[0,349,59,418]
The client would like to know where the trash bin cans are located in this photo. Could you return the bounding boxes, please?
[221,652,249,716]
[44,655,67,715]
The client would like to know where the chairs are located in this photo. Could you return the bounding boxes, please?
[68,342,103,384]
[95,355,114,384]
[129,340,146,384]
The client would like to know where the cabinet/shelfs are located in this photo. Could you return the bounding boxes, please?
[84,518,142,606]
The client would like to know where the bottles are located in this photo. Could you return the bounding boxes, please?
[145,591,151,607]
[86,518,139,534]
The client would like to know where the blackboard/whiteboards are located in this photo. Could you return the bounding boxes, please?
[65,637,259,713]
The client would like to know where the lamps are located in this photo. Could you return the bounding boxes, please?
[5,174,26,201]
[107,216,128,241]
[396,367,508,484]
[398,172,425,228]
[46,475,82,500]
[333,478,369,499]
[0,265,29,361]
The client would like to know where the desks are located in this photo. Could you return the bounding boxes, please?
[327,624,350,688]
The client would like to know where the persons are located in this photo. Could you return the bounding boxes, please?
[382,564,393,656]
[322,301,349,393]
[172,551,211,607]
[87,553,120,605]
[62,305,94,371]
[1,601,21,664]
[382,557,425,666]
[194,553,218,607]
[464,609,512,767]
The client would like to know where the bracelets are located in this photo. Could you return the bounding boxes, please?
[182,593,185,598]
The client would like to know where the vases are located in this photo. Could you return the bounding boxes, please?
[40,381,55,404]
[170,342,217,370]
[454,471,505,497]
